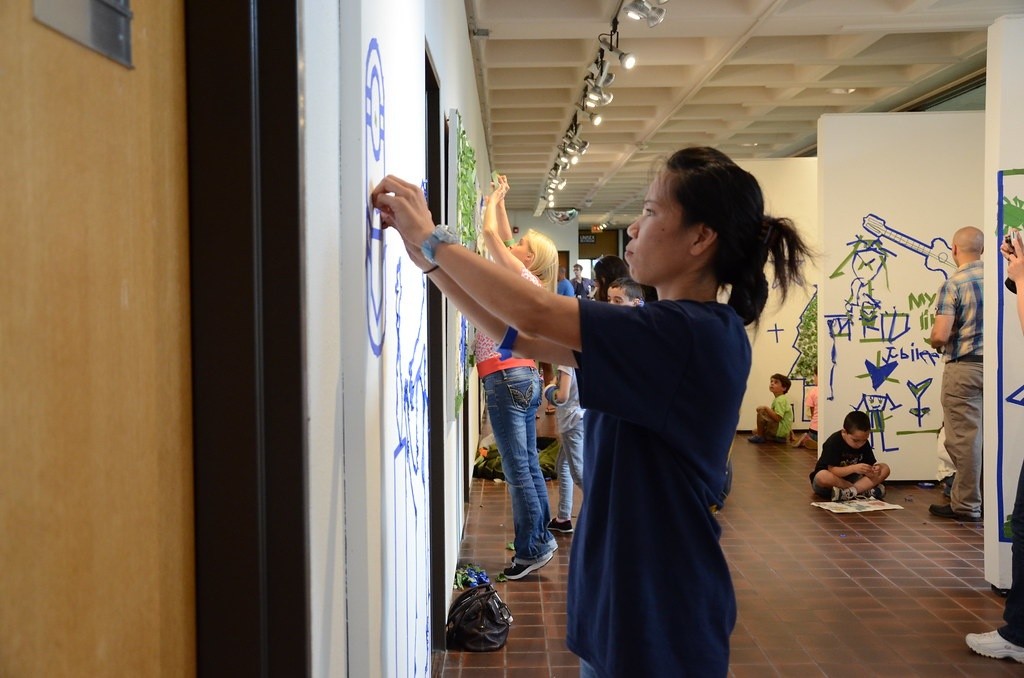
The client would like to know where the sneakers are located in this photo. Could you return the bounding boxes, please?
[511,545,558,562]
[831,486,858,502]
[966,629,1024,662]
[547,518,573,533]
[861,484,885,500]
[504,552,554,580]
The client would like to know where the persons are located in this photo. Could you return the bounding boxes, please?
[809,411,890,501]
[929,225,984,523]
[937,426,956,497]
[966,229,1024,664]
[540,256,645,533]
[748,374,795,443]
[476,175,559,580]
[792,366,818,450]
[371,145,806,678]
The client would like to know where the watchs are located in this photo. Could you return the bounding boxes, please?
[422,225,458,264]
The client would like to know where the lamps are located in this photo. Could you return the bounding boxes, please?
[544,0,666,205]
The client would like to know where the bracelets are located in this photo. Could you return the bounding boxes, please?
[423,265,439,274]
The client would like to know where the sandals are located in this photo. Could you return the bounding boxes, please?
[545,407,555,415]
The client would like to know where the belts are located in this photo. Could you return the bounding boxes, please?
[950,354,983,363]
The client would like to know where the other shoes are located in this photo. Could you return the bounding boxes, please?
[791,432,810,448]
[804,438,818,449]
[748,435,767,444]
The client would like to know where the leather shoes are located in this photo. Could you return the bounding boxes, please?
[929,504,981,521]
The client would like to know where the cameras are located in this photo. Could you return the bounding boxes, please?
[1005,232,1017,248]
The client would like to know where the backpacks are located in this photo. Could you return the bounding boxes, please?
[446,583,514,653]
